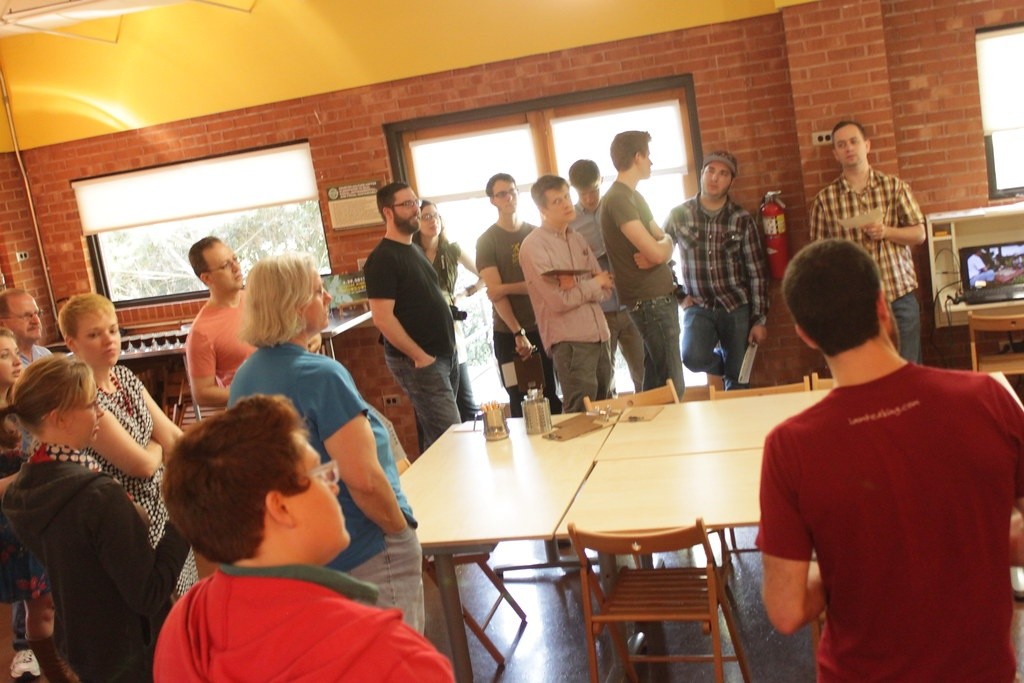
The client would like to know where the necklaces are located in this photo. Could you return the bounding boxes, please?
[99,371,134,418]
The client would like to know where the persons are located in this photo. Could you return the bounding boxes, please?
[412,200,486,422]
[0,327,192,683]
[56,291,199,604]
[599,132,685,404]
[567,160,646,398]
[967,246,994,289]
[519,175,612,414]
[364,183,461,446]
[663,152,769,391]
[153,394,455,683]
[226,255,425,638]
[0,333,78,682]
[475,174,561,418]
[184,237,258,407]
[753,238,1024,683]
[1,286,53,373]
[811,121,927,365]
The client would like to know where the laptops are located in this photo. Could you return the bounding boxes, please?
[958,241,1024,305]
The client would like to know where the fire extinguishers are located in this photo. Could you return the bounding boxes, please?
[759,190,790,278]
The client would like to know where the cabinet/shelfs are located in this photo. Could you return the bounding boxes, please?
[924,201,1024,330]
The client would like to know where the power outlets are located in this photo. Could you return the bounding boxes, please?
[383,393,401,407]
[998,339,1021,353]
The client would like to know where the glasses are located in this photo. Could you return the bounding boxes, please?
[0,311,41,320]
[493,188,517,198]
[299,459,340,485]
[393,198,422,208]
[422,213,440,221]
[212,254,242,271]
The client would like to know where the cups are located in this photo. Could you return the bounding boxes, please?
[520,385,553,435]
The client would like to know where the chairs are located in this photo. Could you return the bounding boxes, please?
[966,309,1024,377]
[812,370,833,389]
[582,376,736,580]
[707,375,811,400]
[567,517,752,683]
[421,554,527,667]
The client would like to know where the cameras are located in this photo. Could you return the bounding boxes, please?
[450,305,467,320]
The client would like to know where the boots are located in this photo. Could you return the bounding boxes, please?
[25,633,80,683]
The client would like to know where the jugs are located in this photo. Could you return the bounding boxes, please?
[474,400,510,441]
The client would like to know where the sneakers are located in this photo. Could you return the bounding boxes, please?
[10,649,41,682]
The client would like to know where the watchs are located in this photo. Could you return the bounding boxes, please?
[513,328,526,339]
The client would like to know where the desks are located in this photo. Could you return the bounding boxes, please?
[318,310,374,359]
[393,387,835,683]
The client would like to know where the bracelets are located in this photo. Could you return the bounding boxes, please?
[466,285,477,296]
